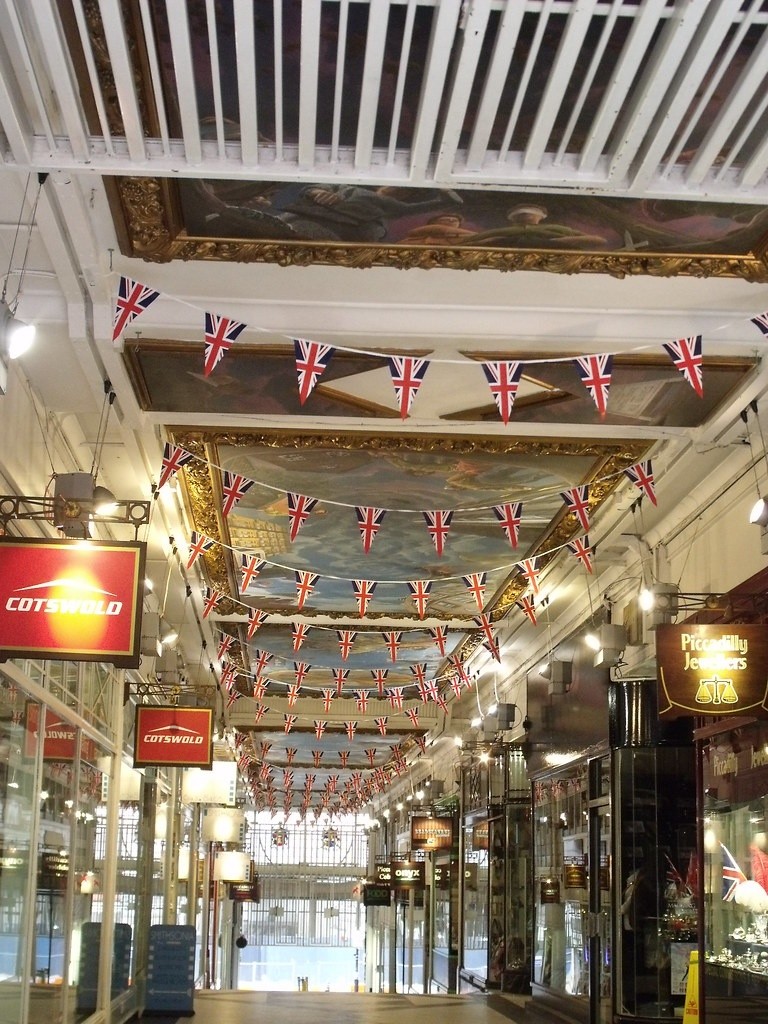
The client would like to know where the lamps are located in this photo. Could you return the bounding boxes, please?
[749,499,768,526]
[584,630,600,650]
[143,585,153,599]
[159,618,177,644]
[6,314,36,360]
[94,486,120,517]
